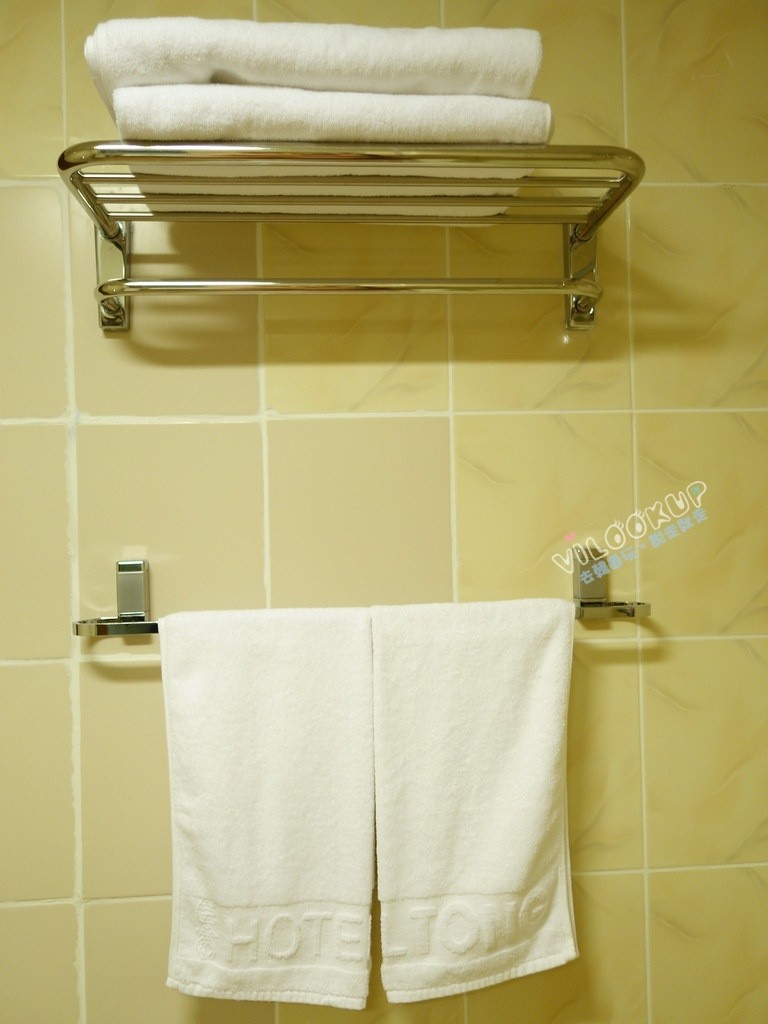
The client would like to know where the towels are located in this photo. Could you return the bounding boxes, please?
[160,605,372,1011]
[82,14,556,228]
[373,599,580,1006]
